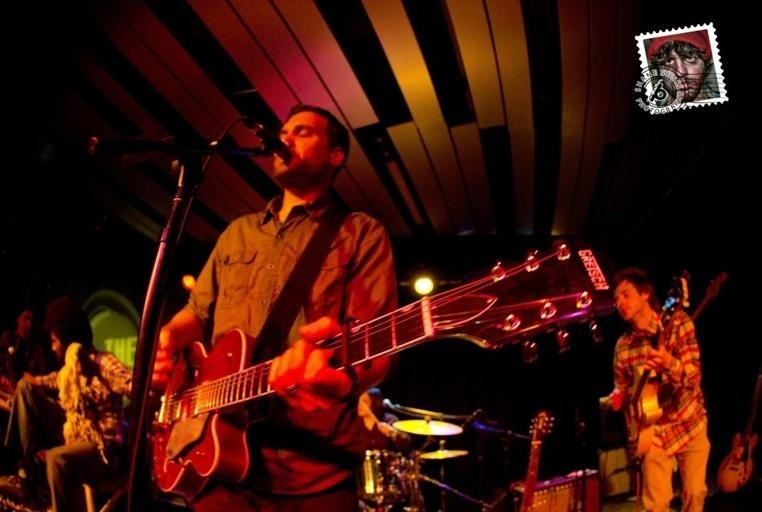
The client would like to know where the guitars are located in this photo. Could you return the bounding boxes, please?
[717,375,761,492]
[154,238,617,500]
[624,272,690,459]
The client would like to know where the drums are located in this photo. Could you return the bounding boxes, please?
[358,450,422,508]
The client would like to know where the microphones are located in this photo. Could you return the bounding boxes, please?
[242,115,290,160]
[382,398,398,412]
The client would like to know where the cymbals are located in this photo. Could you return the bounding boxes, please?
[419,450,468,460]
[392,420,463,436]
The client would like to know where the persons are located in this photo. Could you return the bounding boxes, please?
[644,30,719,107]
[2,308,47,466]
[494,339,570,395]
[357,387,412,451]
[24,299,133,511]
[149,105,397,512]
[599,266,713,511]
